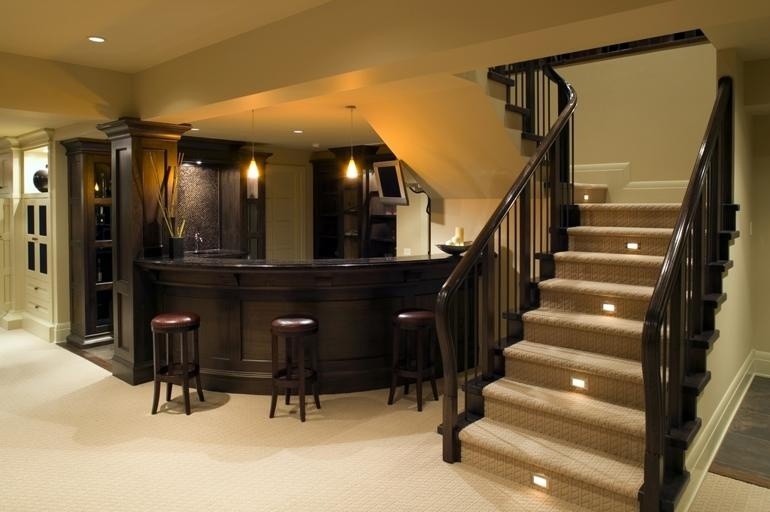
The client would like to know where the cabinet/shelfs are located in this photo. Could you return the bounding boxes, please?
[341,165,397,258]
[57,137,112,348]
[20,142,50,324]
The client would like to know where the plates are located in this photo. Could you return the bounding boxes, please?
[435,241,473,255]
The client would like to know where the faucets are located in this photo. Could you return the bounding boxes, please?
[192,232,203,251]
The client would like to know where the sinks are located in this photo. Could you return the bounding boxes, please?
[192,251,231,254]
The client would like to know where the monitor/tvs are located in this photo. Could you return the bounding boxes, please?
[373,160,409,205]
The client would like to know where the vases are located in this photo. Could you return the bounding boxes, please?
[168,237,184,259]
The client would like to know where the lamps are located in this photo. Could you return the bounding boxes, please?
[246,110,260,179]
[344,106,359,179]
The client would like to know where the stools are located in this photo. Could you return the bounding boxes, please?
[151,310,206,415]
[388,308,439,412]
[269,313,321,422]
